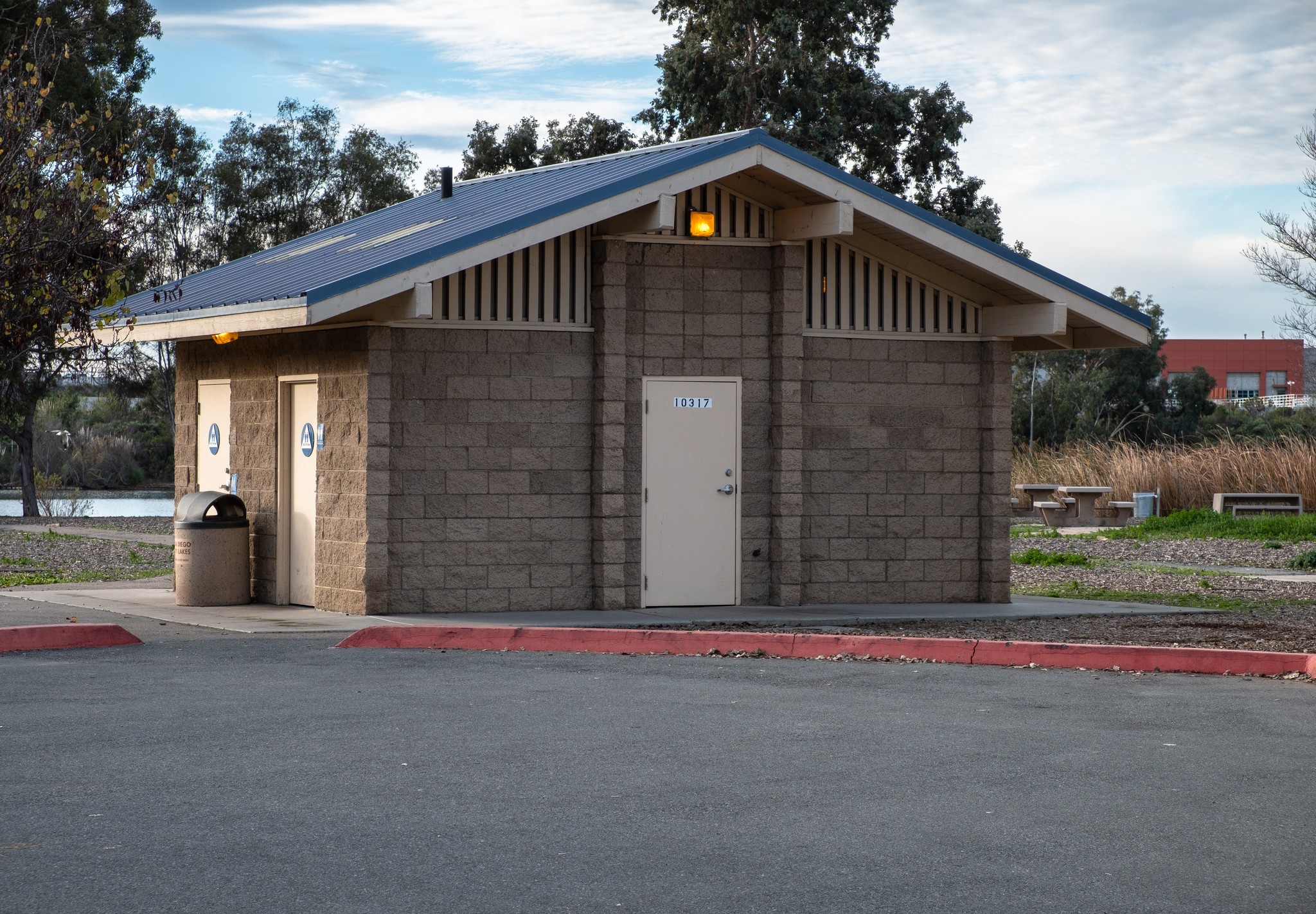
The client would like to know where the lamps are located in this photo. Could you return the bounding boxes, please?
[689,208,714,237]
[212,332,238,344]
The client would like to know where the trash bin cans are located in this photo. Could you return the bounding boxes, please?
[1133,492,1154,518]
[171,490,250,607]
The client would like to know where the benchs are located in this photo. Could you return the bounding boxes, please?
[1011,498,1138,528]
[1224,504,1302,519]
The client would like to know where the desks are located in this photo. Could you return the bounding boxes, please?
[1015,484,1063,517]
[1211,493,1303,519]
[1058,486,1113,526]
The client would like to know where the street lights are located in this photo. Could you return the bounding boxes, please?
[1287,381,1295,395]
